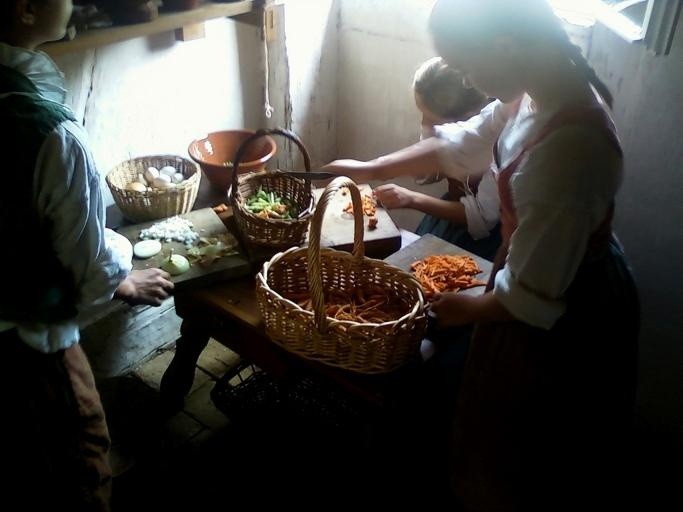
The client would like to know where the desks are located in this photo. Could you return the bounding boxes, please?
[131,224,418,487]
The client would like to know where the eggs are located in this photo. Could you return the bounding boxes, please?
[127,166,184,192]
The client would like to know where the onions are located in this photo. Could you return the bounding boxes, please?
[161,254,190,276]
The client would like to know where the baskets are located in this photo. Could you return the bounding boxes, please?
[105,154,202,225]
[227,128,317,251]
[256,178,430,376]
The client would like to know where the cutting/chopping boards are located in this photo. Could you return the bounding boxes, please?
[114,206,253,295]
[296,184,401,252]
[380,232,494,364]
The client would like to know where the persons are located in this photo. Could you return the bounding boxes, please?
[312,1,641,512]
[372,61,502,265]
[0,1,176,512]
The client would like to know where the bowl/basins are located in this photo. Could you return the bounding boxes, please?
[187,128,276,196]
